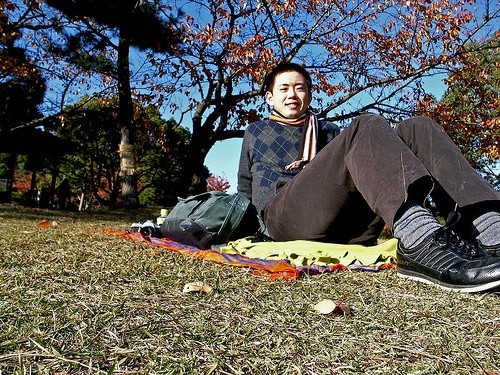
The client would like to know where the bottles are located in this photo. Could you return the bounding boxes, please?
[157,209,169,225]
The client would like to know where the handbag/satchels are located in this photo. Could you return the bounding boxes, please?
[159,190,250,251]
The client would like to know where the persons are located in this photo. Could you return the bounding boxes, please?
[237,62,500,292]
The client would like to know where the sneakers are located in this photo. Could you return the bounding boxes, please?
[396,211,500,293]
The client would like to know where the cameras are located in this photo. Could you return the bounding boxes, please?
[132,225,162,238]
